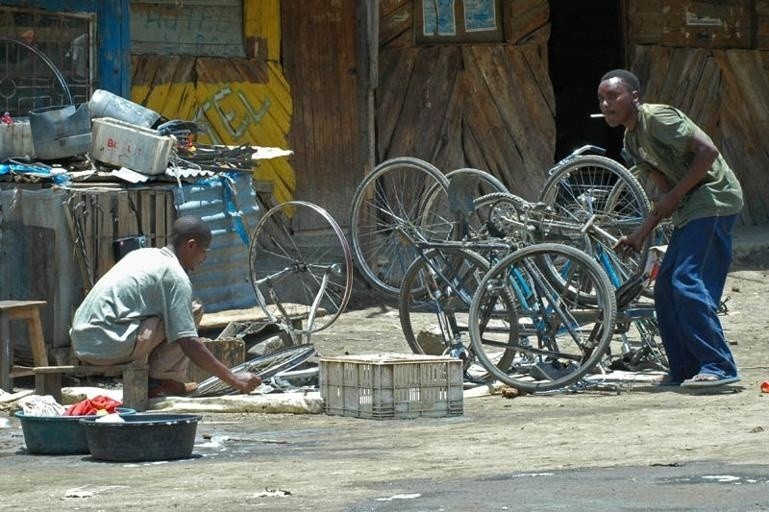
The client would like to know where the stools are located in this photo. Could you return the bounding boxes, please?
[32,365,149,410]
[0,298,47,395]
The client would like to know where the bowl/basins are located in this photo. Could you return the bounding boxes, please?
[78,413,204,459]
[14,405,137,456]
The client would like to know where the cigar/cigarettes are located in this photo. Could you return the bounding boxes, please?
[590,113,608,118]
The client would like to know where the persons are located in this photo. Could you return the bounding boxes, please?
[597,68,745,390]
[69,215,261,397]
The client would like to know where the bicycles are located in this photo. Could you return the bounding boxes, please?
[185,198,356,396]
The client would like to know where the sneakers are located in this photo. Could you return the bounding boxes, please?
[149,379,199,396]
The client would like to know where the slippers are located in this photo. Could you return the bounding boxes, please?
[678,375,740,388]
[653,374,681,386]
